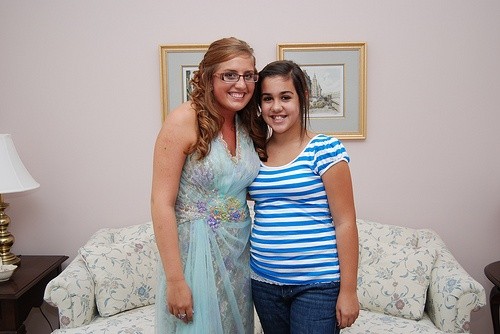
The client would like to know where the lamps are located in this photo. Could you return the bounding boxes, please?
[0,134,40,266]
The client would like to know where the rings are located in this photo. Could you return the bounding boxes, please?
[178,314,186,319]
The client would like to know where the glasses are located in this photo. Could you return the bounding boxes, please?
[213,71,259,84]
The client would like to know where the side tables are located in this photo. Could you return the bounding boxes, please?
[484,261,500,334]
[0,255,69,334]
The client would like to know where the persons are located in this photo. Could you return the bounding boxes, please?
[151,37,269,334]
[247,59,360,334]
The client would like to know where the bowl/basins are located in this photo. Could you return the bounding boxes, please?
[0,265,18,282]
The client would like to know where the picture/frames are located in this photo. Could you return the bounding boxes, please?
[159,44,209,125]
[275,42,366,141]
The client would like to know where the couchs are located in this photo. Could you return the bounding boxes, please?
[43,222,487,334]
